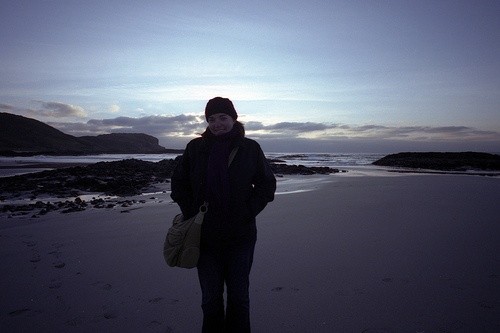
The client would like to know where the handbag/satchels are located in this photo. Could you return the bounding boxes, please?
[163,213,204,268]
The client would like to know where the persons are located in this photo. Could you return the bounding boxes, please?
[170,97,276,333]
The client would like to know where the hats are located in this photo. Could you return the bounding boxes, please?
[205,97,237,121]
